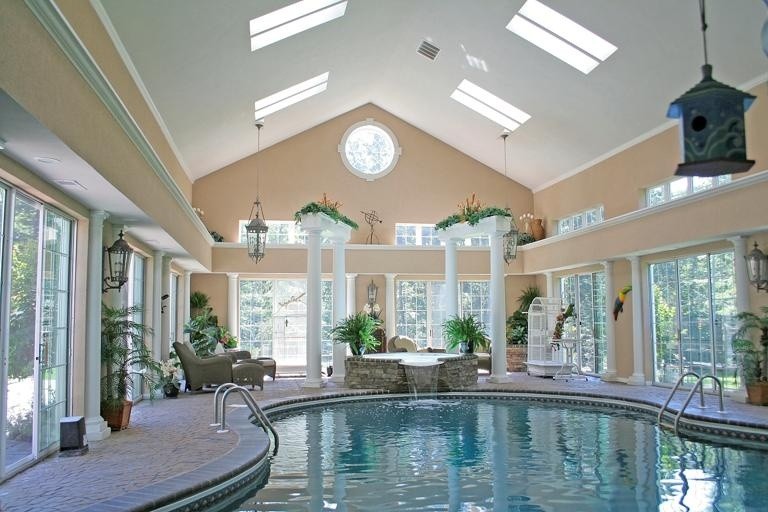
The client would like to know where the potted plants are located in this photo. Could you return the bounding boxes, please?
[292,202,338,232]
[728,304,767,408]
[443,310,489,353]
[504,308,527,373]
[326,311,382,357]
[432,211,464,242]
[328,215,359,243]
[458,191,512,236]
[103,306,173,431]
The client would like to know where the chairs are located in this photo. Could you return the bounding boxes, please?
[386,336,445,353]
[184,341,251,360]
[469,337,491,374]
[171,340,232,393]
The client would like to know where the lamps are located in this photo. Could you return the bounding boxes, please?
[500,134,518,264]
[103,229,131,296]
[741,240,767,294]
[366,277,378,317]
[244,123,268,266]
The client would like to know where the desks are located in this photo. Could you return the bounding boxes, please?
[548,338,588,383]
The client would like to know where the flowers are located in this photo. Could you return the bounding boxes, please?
[159,356,179,384]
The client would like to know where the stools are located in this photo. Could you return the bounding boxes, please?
[232,364,263,391]
[235,358,276,381]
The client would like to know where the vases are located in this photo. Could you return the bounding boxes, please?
[164,382,180,398]
[533,219,544,240]
